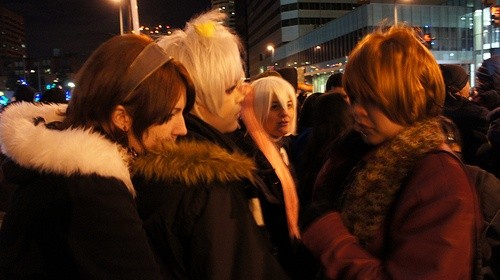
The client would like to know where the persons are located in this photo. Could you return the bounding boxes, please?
[236,75,303,239]
[134,8,289,280]
[438,48,500,280]
[300,21,477,280]
[14,71,350,193]
[0,33,196,280]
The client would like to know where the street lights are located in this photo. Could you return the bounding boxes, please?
[393,0,408,24]
[114,1,125,35]
[266,44,275,66]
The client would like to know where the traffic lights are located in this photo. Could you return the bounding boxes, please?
[424,33,432,50]
[490,6,500,27]
